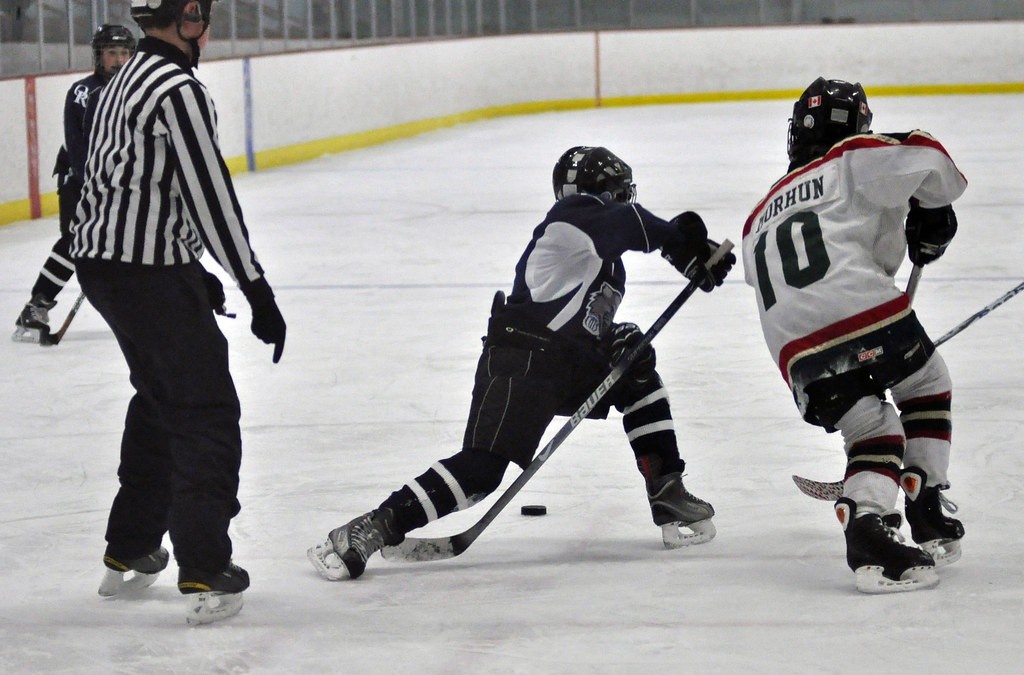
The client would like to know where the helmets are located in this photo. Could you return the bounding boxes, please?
[553,145,637,207]
[91,24,137,81]
[129,0,219,28]
[788,76,875,165]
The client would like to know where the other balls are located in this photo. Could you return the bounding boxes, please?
[521,505,546,516]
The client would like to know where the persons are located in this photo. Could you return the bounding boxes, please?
[16,23,136,331]
[741,76,968,581]
[69,0,287,594]
[328,145,737,578]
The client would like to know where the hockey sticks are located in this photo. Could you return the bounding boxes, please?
[935,286,1020,348]
[793,264,920,502]
[37,292,83,345]
[381,239,735,562]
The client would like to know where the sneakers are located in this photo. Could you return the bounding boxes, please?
[635,456,716,547]
[97,541,169,597]
[177,563,250,624]
[12,294,58,343]
[899,465,965,567]
[308,506,396,582]
[832,497,940,594]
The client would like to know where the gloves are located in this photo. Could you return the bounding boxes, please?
[203,271,227,315]
[242,279,287,363]
[905,197,957,268]
[657,211,737,292]
[607,321,654,383]
